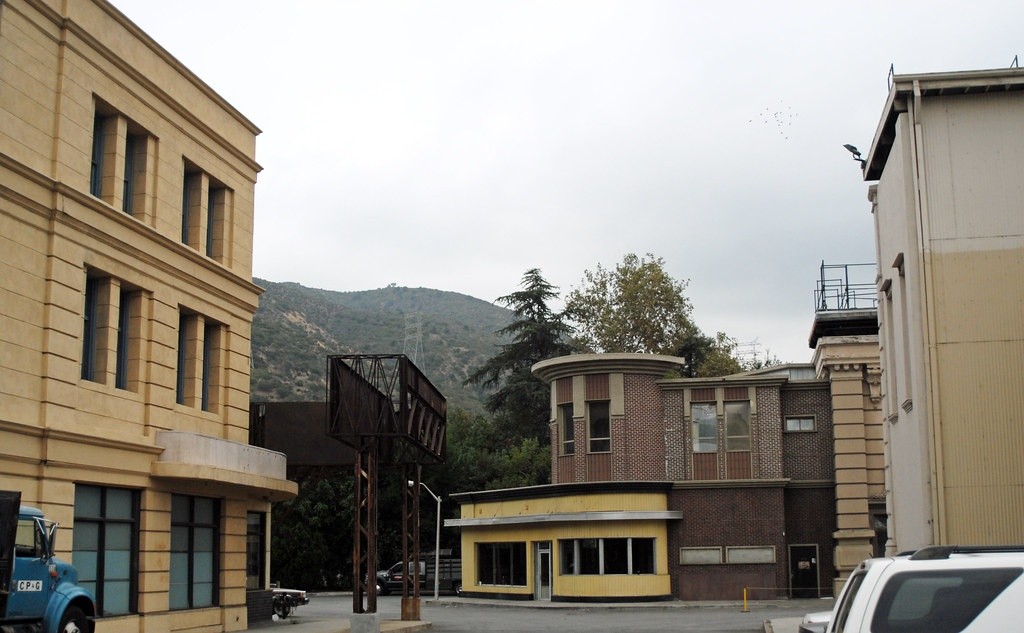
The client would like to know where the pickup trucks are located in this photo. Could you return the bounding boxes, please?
[366,558,464,597]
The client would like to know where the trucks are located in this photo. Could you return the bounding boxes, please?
[1,488,96,633]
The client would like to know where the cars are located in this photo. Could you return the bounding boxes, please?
[269,587,308,620]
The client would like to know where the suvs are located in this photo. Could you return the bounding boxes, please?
[823,542,1024,632]
